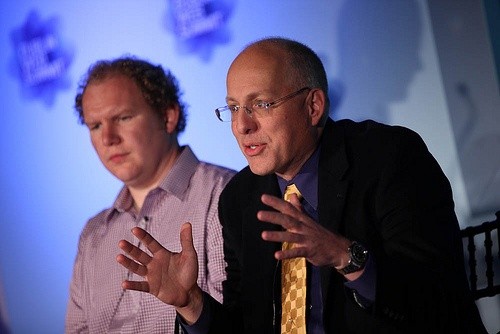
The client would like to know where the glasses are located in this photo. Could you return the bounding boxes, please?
[215,87,311,122]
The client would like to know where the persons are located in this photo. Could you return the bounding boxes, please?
[115,37,489,334]
[64,55,239,334]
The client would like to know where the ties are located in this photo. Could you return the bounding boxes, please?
[281,184,306,334]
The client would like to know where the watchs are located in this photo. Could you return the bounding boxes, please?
[336,239,369,276]
